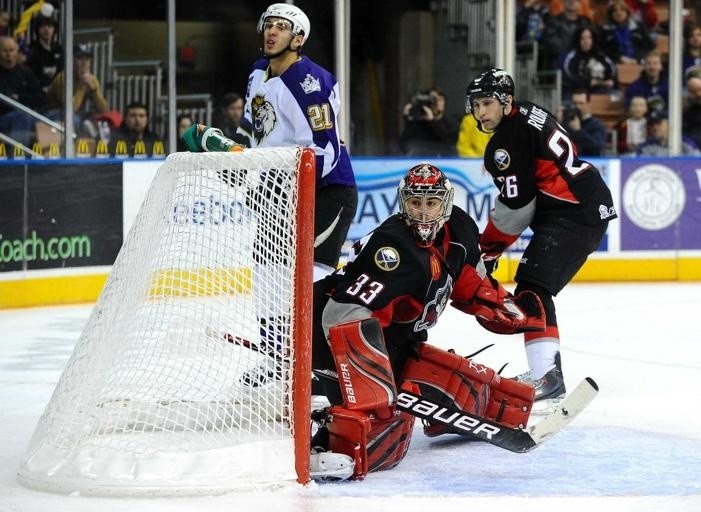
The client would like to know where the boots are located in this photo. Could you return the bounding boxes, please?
[509,350,566,403]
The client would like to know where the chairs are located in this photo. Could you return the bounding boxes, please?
[0,41,81,156]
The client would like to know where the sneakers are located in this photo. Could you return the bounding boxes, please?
[238,358,289,388]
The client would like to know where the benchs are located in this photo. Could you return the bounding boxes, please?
[523,0,696,140]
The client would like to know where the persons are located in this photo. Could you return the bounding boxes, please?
[216,3,358,386]
[292,165,548,481]
[400,89,453,158]
[1,1,243,156]
[515,1,700,157]
[467,68,617,404]
[456,112,495,159]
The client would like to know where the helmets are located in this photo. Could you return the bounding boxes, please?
[396,164,454,246]
[256,2,310,47]
[463,68,514,114]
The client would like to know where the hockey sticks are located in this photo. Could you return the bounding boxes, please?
[206,326,598,454]
